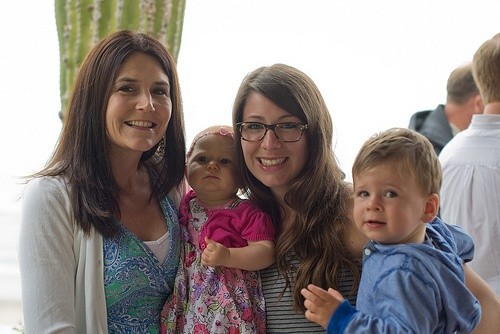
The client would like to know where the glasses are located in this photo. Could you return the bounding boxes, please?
[235,121,309,144]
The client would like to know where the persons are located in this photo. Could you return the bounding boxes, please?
[437,33,500,300]
[232,63,500,334]
[14,30,187,334]
[301,127,482,334]
[161,125,277,334]
[409,62,485,157]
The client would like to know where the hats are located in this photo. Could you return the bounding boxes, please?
[186,116,238,166]
